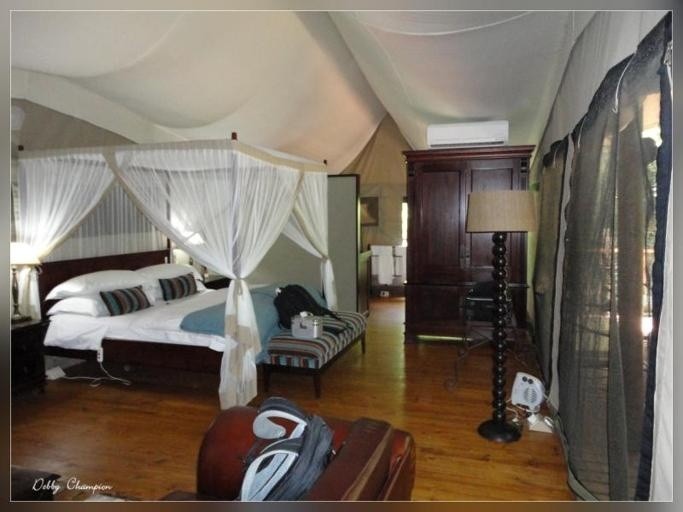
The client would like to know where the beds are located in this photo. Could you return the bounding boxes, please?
[10,130,341,412]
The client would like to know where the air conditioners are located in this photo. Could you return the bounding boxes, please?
[424,118,512,150]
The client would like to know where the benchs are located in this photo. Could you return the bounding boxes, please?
[257,307,368,400]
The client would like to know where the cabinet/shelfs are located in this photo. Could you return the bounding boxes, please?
[402,140,539,338]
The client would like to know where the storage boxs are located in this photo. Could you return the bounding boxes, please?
[290,311,326,342]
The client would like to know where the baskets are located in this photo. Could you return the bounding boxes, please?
[291,311,322,339]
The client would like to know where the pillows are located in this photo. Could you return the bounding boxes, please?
[42,261,210,319]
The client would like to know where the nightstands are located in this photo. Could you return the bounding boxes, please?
[201,274,232,291]
[10,317,53,399]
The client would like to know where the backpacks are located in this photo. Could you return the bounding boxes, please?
[237,396,334,502]
[274,285,349,334]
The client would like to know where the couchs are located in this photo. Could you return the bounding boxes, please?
[156,401,422,503]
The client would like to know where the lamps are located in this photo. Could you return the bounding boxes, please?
[465,187,542,445]
[10,239,40,325]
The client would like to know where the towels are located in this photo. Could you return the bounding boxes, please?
[370,242,407,287]
